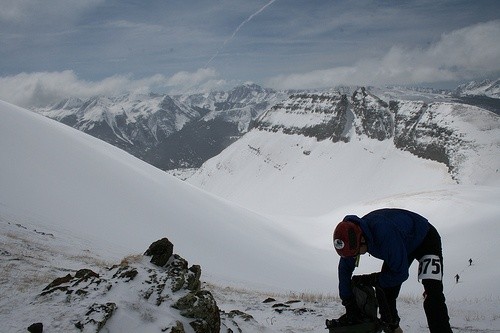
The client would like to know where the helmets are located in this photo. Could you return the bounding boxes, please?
[332,219,362,258]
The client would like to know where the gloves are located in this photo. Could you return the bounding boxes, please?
[352,274,376,286]
[345,300,365,324]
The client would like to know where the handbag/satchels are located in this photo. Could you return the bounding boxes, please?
[325,282,383,333]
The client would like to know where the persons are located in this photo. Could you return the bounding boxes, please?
[455,274,460,283]
[332,208,454,333]
[469,257,472,265]
